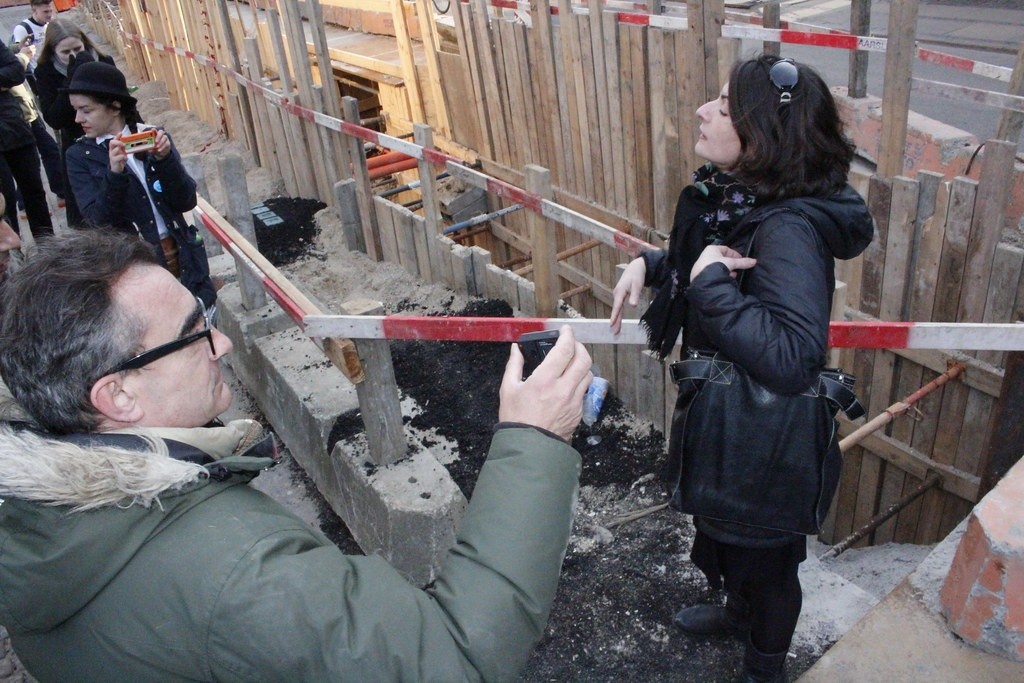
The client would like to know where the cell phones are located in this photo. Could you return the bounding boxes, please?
[120,131,156,154]
[29,34,34,46]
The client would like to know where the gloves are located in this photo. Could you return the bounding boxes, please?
[64,52,94,86]
[98,54,116,66]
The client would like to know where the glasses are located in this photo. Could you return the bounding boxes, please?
[101,297,217,377]
[769,58,798,104]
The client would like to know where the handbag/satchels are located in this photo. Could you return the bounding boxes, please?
[665,209,867,535]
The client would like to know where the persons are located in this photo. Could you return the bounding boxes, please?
[0,1,218,315]
[0,231,592,683]
[607,55,879,681]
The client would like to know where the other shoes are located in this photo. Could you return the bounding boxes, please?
[56,199,66,208]
[20,211,51,220]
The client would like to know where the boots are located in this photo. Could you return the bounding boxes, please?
[674,603,732,632]
[737,628,786,683]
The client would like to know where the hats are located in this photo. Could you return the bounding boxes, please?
[57,62,137,102]
